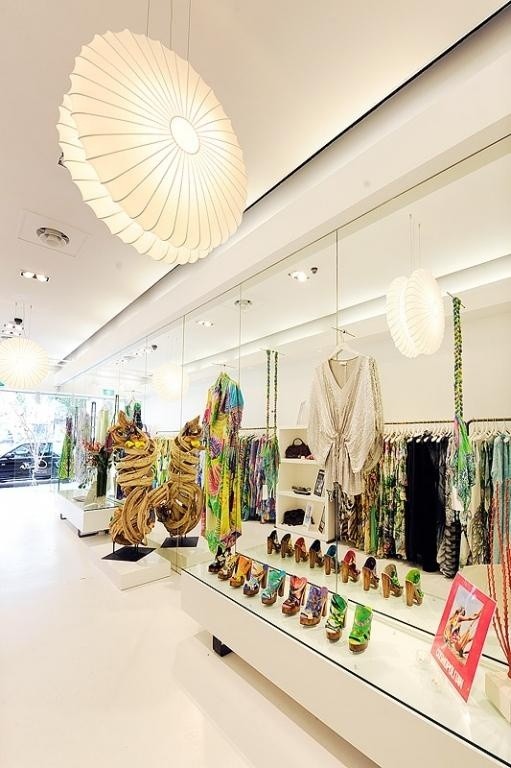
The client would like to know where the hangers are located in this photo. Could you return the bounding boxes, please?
[328,330,360,364]
[382,415,511,443]
[238,426,275,441]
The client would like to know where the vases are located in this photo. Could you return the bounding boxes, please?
[96,463,108,497]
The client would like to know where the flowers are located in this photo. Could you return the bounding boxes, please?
[78,437,113,490]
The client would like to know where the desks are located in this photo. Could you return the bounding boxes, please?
[57,489,124,539]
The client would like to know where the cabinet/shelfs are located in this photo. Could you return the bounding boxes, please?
[178,534,511,767]
[274,425,336,543]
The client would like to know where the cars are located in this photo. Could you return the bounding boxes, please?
[0,442,61,480]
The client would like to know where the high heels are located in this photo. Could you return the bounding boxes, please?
[300,585,328,626]
[243,563,269,596]
[294,537,309,563]
[341,550,361,584]
[218,554,240,580]
[325,594,349,641]
[309,539,324,569]
[404,568,425,607]
[363,557,380,592]
[324,545,341,575]
[230,556,252,588]
[208,546,232,573]
[267,529,282,554]
[382,564,404,598]
[349,605,374,653]
[282,576,309,615]
[281,533,294,559]
[262,569,287,605]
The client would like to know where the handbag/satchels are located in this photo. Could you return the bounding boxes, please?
[283,509,315,527]
[285,437,311,459]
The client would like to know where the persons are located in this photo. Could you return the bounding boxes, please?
[443,605,482,659]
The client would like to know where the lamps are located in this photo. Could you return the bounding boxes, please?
[17,211,85,257]
[57,0,248,267]
[0,299,32,340]
[385,213,444,360]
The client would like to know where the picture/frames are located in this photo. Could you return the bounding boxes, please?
[430,573,497,706]
